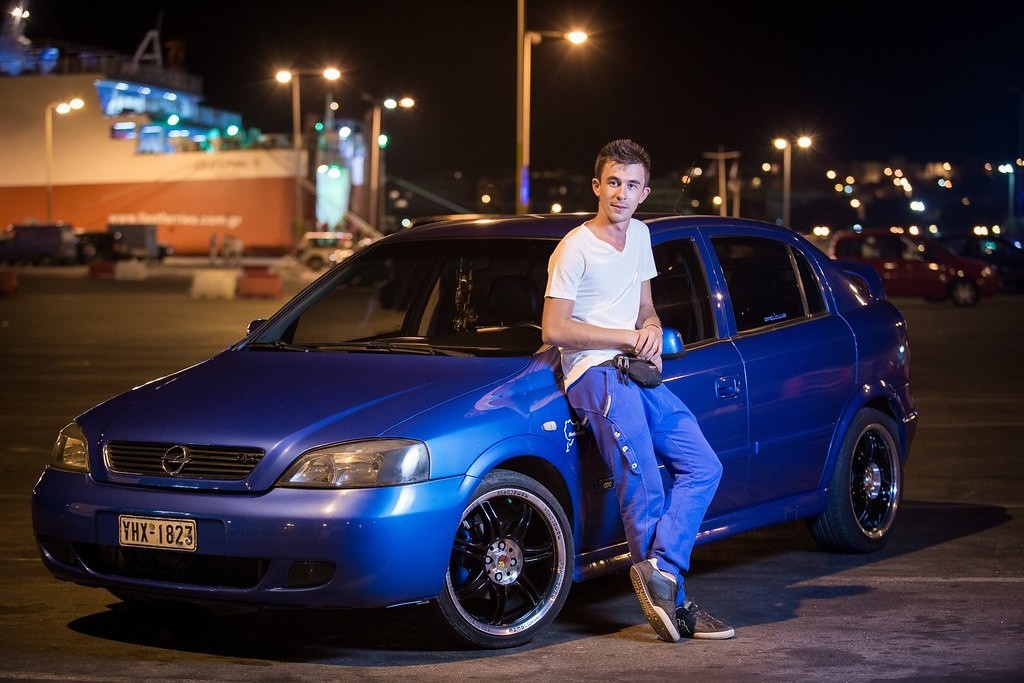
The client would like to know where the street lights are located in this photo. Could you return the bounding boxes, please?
[371,98,415,227]
[275,67,340,243]
[704,151,741,216]
[775,137,812,228]
[46,98,84,224]
[516,31,586,215]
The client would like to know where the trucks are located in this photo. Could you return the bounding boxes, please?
[107,224,175,262]
[0,223,78,267]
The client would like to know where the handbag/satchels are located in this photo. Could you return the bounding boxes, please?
[613,352,662,387]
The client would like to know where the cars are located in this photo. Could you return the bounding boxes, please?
[31,211,919,648]
[75,233,123,266]
[927,234,1024,295]
[296,232,353,273]
[829,228,1000,308]
[379,215,491,309]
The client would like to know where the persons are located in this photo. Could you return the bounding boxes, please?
[541,139,735,642]
[208,231,242,267]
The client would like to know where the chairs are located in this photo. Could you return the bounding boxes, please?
[484,272,537,326]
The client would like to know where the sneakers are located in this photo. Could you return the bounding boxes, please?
[630,557,681,641]
[671,600,736,639]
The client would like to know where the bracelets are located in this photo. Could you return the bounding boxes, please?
[644,323,663,335]
